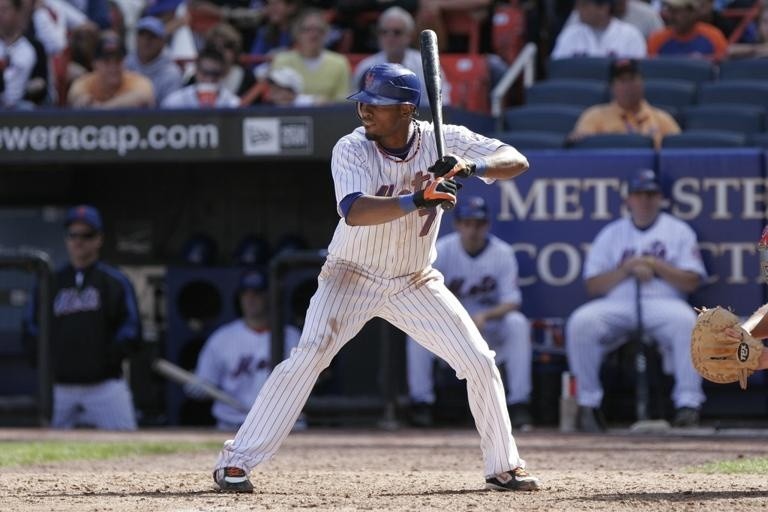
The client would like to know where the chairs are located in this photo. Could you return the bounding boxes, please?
[334,0,768,163]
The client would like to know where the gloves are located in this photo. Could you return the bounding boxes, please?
[415,177,467,210]
[426,150,477,175]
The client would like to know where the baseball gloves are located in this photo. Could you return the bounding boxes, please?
[690,305,764,389]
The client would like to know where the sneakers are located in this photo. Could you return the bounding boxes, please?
[482,461,541,494]
[211,449,256,496]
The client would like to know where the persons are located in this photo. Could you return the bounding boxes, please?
[1,1,575,109]
[394,192,535,433]
[212,70,540,490]
[25,201,143,430]
[182,265,309,433]
[565,58,683,147]
[563,165,709,428]
[549,1,767,62]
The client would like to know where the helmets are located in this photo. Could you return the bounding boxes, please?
[345,63,421,112]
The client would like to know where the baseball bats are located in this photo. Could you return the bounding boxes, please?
[155,359,249,415]
[422,29,454,211]
[635,281,648,421]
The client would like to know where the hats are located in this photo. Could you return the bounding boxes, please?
[61,204,106,231]
[264,67,305,95]
[91,29,128,61]
[133,14,169,41]
[607,57,645,86]
[454,195,491,224]
[236,268,270,293]
[626,165,665,194]
[196,48,230,77]
